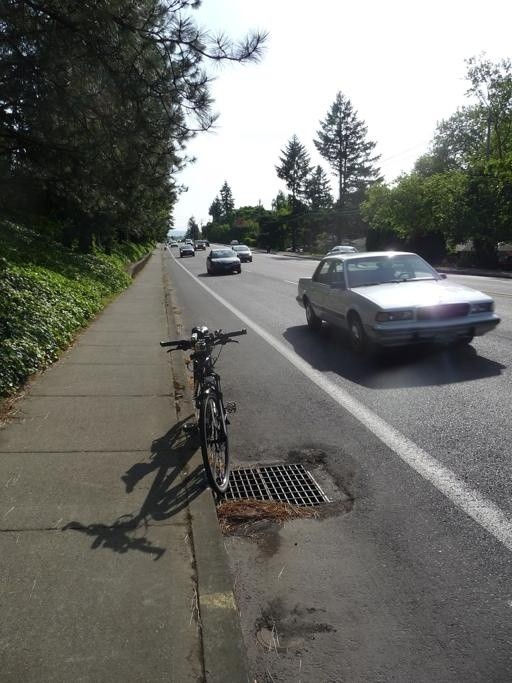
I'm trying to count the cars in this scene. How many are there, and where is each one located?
[326,244,360,267]
[295,250,499,356]
[232,240,239,245]
[232,244,252,263]
[205,248,241,275]
[168,237,210,258]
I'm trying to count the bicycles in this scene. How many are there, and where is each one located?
[159,324,248,493]
